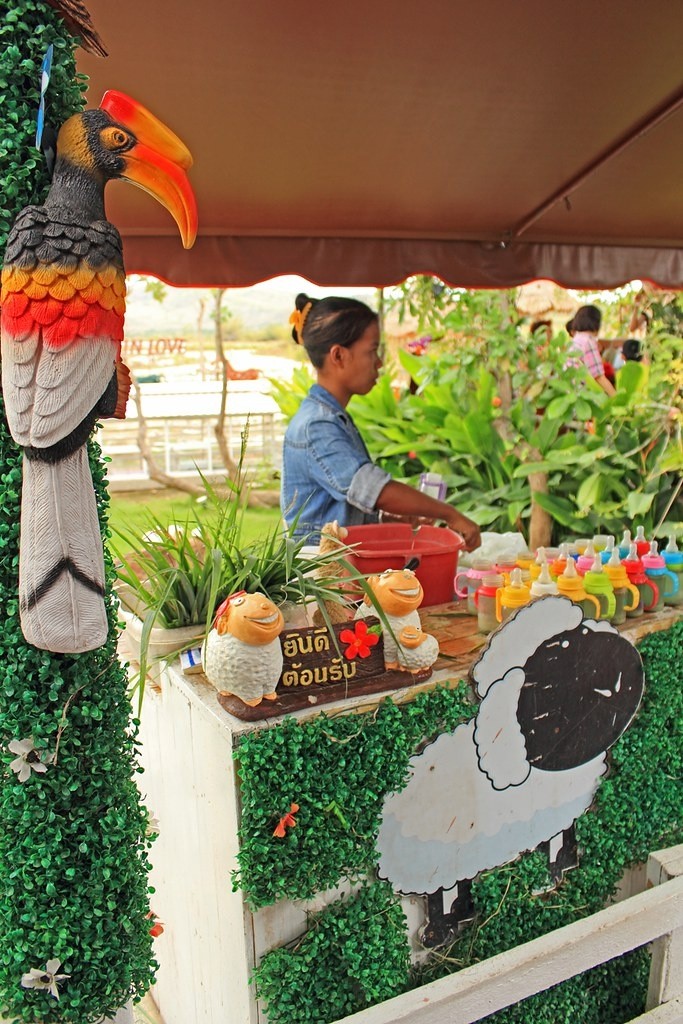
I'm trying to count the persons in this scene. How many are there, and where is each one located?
[281,293,482,583]
[530,305,641,432]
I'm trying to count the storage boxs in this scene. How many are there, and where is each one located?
[110,596,291,689]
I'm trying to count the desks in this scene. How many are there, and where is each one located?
[148,602,683,1023]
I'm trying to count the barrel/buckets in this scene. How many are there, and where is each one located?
[335,520,467,616]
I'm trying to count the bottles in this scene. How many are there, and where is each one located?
[453,526,683,637]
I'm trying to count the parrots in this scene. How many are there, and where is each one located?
[0,88,198,655]
